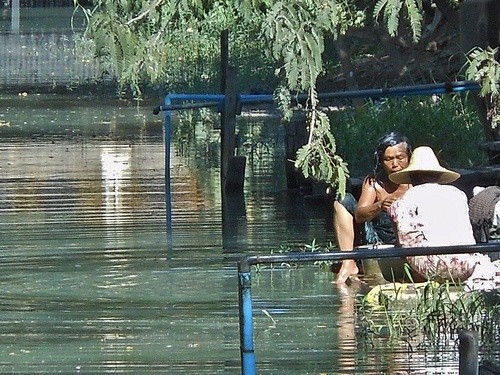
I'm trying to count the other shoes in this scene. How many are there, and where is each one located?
[331,261,364,275]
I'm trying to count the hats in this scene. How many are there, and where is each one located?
[389,146,461,184]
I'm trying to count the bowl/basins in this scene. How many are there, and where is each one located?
[375,257,400,282]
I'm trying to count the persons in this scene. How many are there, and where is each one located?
[387,146,478,283]
[332,131,413,283]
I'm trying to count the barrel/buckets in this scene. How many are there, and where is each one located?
[354,242,396,278]
